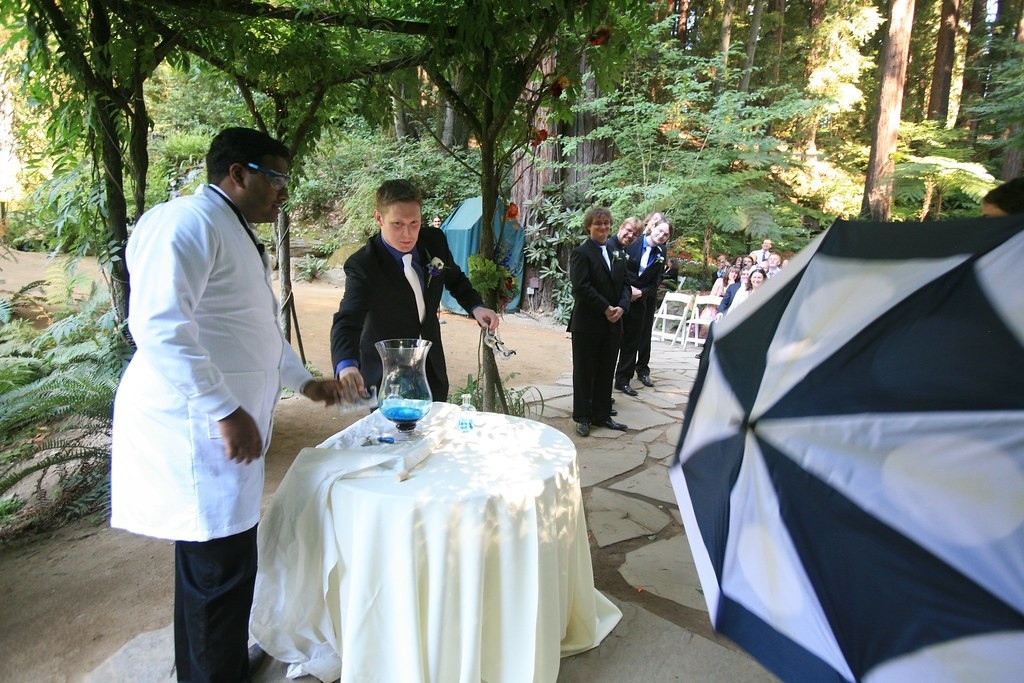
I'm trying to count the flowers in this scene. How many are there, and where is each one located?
[493,244,525,321]
[549,70,589,104]
[528,127,551,146]
[612,249,630,266]
[588,23,613,48]
[423,247,451,288]
[654,254,664,264]
[502,198,520,223]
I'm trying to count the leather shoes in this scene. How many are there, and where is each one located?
[638,375,655,387]
[615,385,638,396]
[576,421,590,436]
[591,418,627,430]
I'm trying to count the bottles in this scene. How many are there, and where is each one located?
[385,385,405,409]
[456,394,476,433]
[374,339,432,440]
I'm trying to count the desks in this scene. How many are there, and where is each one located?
[244,398,624,683]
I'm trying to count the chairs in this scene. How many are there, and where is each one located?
[649,291,723,350]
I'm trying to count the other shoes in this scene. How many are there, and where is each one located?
[248,644,265,676]
[609,410,617,415]
[612,398,615,403]
[695,354,700,359]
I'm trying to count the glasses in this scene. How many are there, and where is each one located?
[592,220,609,226]
[249,164,292,190]
[717,260,724,263]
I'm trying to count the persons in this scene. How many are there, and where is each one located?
[979,177,1023,214]
[331,179,499,416]
[695,239,789,358]
[111,127,345,682]
[606,217,645,415]
[433,215,443,228]
[638,212,665,237]
[658,257,680,310]
[625,219,673,386]
[567,207,628,436]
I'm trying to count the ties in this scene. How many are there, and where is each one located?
[762,252,765,261]
[638,246,651,277]
[601,245,611,269]
[622,246,625,253]
[402,254,426,323]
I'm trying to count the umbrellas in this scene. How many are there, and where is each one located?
[669,214,1024,683]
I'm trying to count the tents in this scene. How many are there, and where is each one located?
[440,195,525,317]
